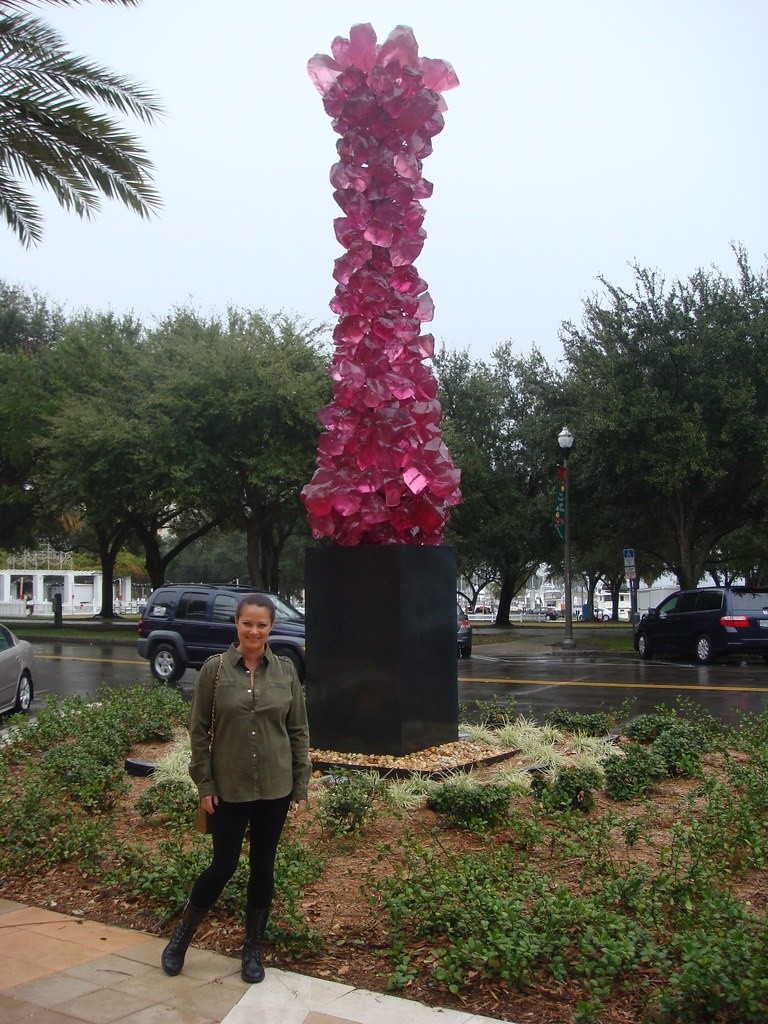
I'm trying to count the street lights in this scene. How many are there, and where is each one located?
[559,427,576,649]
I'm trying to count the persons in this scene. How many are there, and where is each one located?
[161,594,309,983]
[26,594,34,616]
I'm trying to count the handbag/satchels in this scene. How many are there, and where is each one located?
[196,802,211,833]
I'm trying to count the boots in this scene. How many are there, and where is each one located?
[241,919,265,983]
[161,919,197,976]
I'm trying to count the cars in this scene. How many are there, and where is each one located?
[466,606,492,615]
[578,608,614,620]
[1,622,32,715]
[457,602,472,657]
[545,610,565,620]
[635,586,768,662]
[137,583,305,683]
[533,606,550,613]
